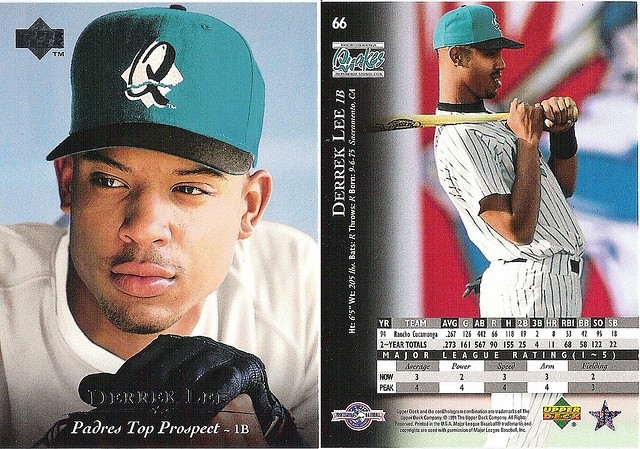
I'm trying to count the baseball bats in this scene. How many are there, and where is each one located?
[344,106,578,135]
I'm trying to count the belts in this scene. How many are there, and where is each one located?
[506,257,580,274]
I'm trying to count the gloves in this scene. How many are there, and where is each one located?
[79,334,310,447]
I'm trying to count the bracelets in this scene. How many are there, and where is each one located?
[549,124,578,159]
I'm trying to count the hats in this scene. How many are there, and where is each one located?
[433,4,525,50]
[46,4,266,176]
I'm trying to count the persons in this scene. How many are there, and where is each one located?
[0,5,320,449]
[431,4,586,319]
